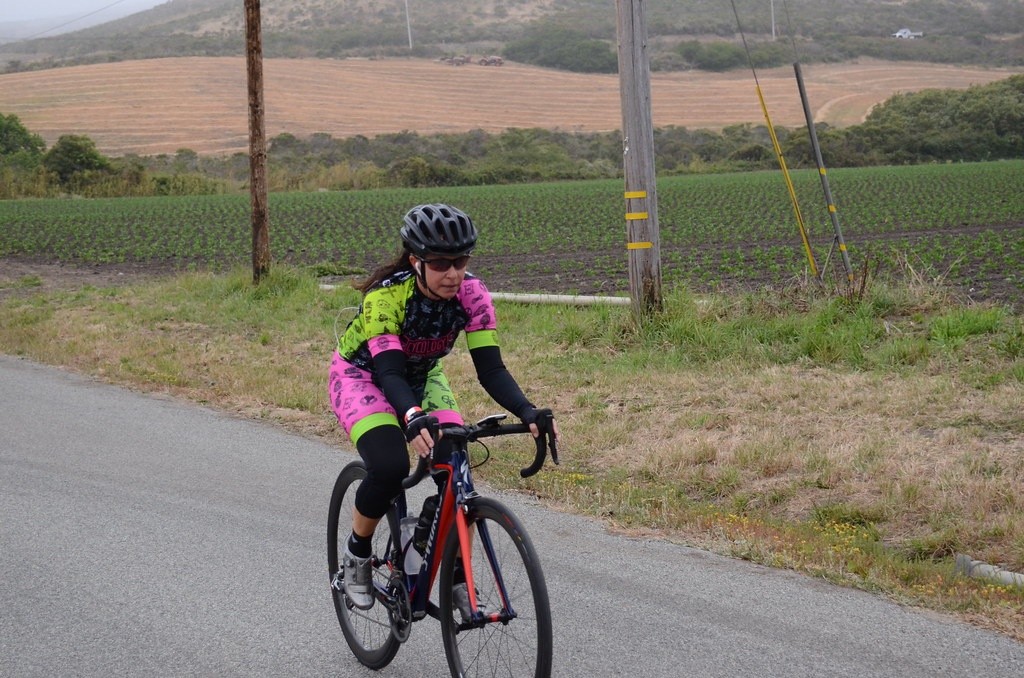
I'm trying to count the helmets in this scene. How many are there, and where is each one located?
[398,203,477,256]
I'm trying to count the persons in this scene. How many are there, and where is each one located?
[328,201,561,630]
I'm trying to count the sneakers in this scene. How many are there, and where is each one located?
[344,533,374,609]
[452,582,486,623]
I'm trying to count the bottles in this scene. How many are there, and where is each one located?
[412,492,439,552]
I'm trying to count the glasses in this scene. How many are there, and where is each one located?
[409,251,471,272]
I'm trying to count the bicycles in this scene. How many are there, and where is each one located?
[322,406,557,677]
